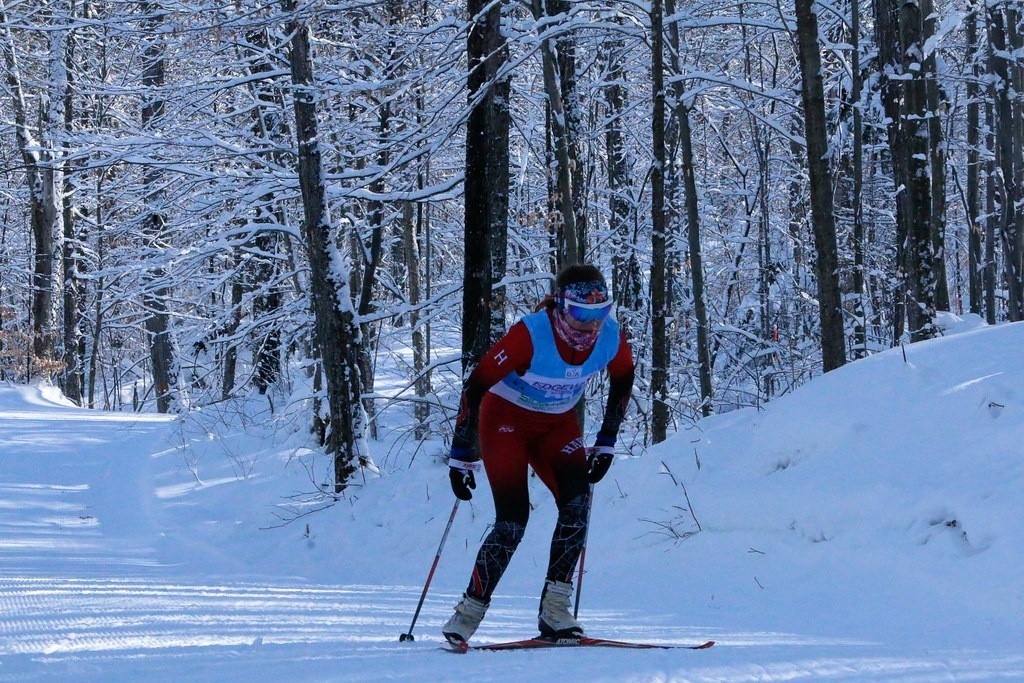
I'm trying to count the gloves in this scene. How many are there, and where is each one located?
[449,467,477,500]
[588,440,614,483]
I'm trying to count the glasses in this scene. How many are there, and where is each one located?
[564,295,614,323]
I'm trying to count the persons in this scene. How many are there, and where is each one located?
[441,263,635,645]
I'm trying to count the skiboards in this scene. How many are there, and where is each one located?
[437,634,719,654]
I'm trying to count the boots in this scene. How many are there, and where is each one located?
[538,577,584,635]
[442,587,491,646]
